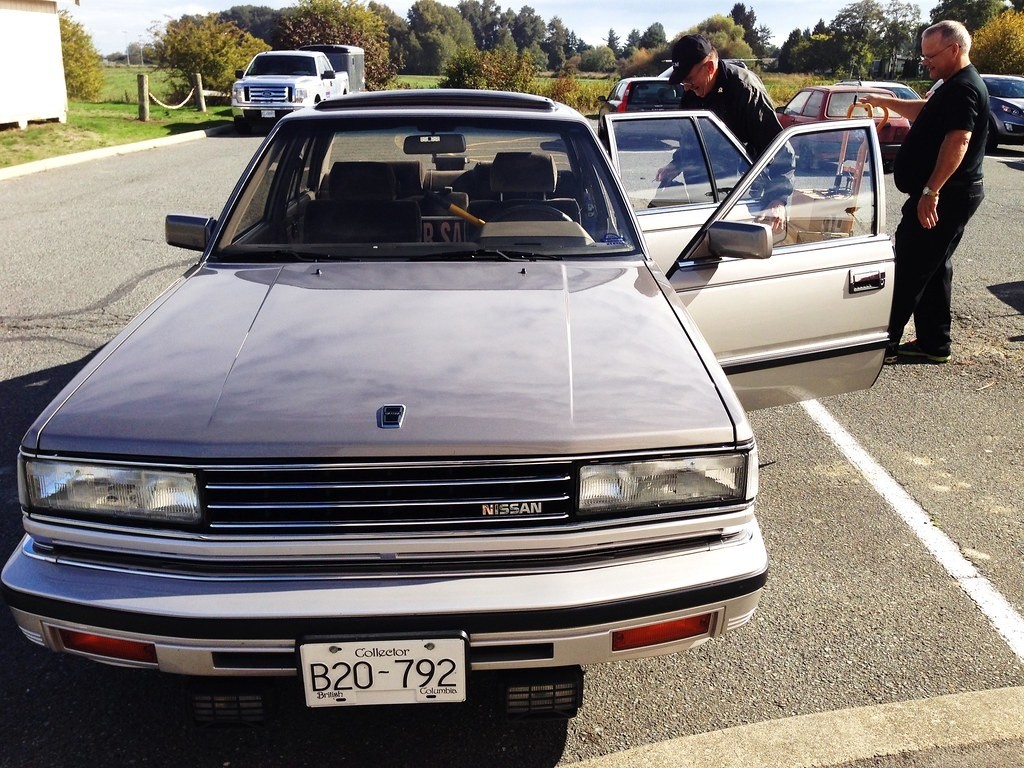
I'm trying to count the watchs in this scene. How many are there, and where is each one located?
[923,187,939,197]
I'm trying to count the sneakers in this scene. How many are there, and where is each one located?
[883,355,897,363]
[897,338,952,361]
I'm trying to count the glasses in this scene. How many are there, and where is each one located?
[920,44,962,62]
[679,63,706,86]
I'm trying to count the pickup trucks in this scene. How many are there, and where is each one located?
[233,50,349,135]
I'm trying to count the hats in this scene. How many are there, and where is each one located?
[668,34,711,85]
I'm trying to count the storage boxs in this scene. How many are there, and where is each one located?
[787,188,853,245]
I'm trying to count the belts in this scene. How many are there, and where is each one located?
[944,180,982,188]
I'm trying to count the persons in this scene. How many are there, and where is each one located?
[655,34,796,236]
[858,20,989,364]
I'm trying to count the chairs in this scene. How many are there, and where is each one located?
[303,151,597,243]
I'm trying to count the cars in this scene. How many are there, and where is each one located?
[0,88,901,725]
[596,77,691,143]
[832,81,923,126]
[775,86,910,174]
[927,75,1024,151]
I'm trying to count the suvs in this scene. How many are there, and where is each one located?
[657,61,750,83]
